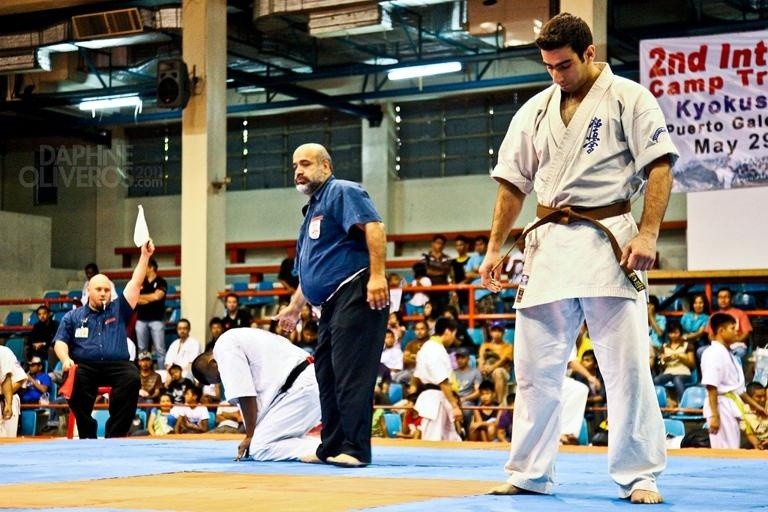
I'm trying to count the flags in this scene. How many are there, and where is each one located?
[132,203,151,248]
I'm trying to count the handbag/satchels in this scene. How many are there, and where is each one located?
[752,342,768,387]
[38,392,50,416]
[146,409,174,435]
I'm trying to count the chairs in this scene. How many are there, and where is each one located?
[227,283,249,306]
[65,290,84,308]
[34,286,61,312]
[19,306,43,336]
[464,324,485,346]
[252,281,279,309]
[18,410,39,437]
[90,408,109,436]
[383,411,404,436]
[160,284,179,305]
[0,308,27,334]
[162,308,184,331]
[399,327,415,348]
[4,337,26,363]
[387,381,406,406]
[505,326,516,350]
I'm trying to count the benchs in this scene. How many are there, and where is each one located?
[116,242,180,264]
[388,223,522,251]
[224,237,297,259]
[93,262,181,282]
[225,261,287,279]
[382,255,427,274]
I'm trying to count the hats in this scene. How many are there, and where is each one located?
[490,320,505,333]
[138,351,153,361]
[28,356,42,364]
[454,347,470,357]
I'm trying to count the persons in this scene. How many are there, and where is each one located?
[275,143,390,469]
[189,326,322,462]
[480,11,684,506]
[1,232,768,450]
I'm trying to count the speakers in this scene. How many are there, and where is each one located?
[155,58,192,108]
